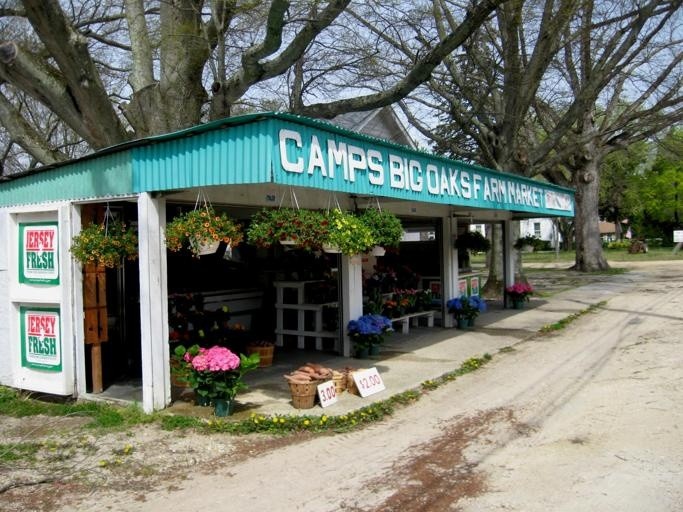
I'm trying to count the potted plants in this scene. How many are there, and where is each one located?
[453,231,545,256]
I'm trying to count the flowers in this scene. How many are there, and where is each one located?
[67,222,138,270]
[506,283,533,303]
[165,209,402,260]
[385,287,436,312]
[169,344,259,398]
[346,313,393,347]
[445,296,486,318]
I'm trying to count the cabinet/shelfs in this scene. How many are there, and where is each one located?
[274,279,436,351]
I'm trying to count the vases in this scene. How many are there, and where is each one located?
[353,345,381,360]
[513,301,522,309]
[458,317,476,328]
[194,388,235,416]
